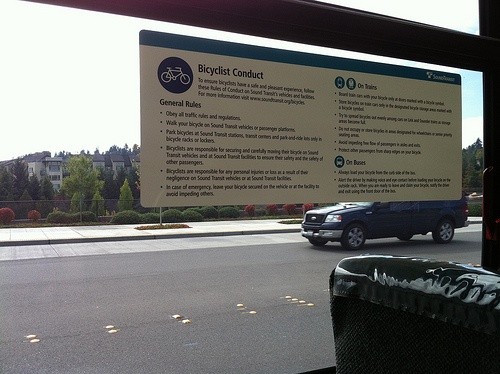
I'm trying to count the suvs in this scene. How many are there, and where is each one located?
[301,194,470,252]
